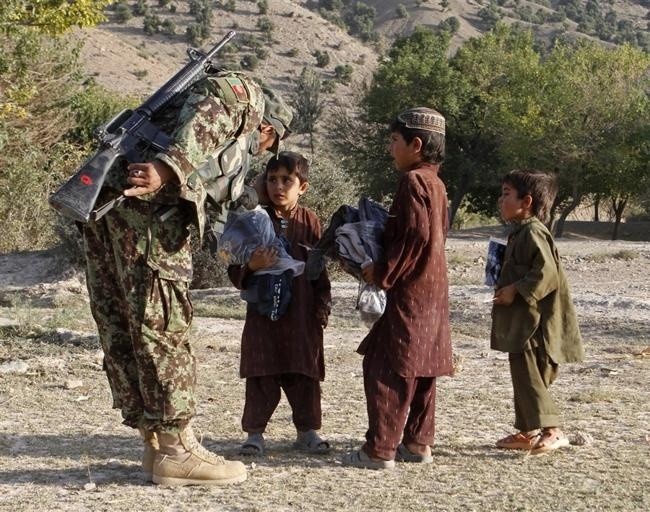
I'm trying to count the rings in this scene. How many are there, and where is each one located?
[133,170,141,177]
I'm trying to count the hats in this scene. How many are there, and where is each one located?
[398,107,445,137]
[260,87,294,160]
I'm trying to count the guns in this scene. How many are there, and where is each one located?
[47,28,237,224]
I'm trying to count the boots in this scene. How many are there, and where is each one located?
[152,426,247,486]
[141,431,160,481]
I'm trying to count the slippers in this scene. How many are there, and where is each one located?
[531,431,569,453]
[241,437,264,455]
[395,444,434,463]
[496,433,541,448]
[293,435,331,454]
[342,452,395,469]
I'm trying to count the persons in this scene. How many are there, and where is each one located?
[323,106,454,471]
[79,71,294,488]
[489,168,586,453]
[226,150,334,457]
